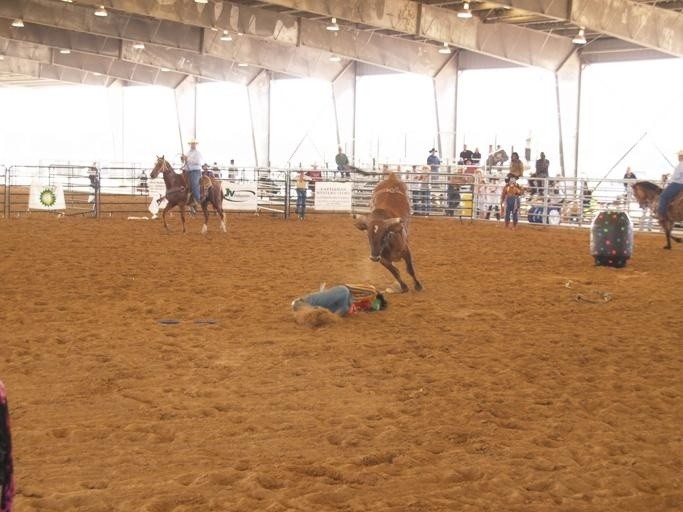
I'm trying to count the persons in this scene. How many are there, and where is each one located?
[380,142,592,228]
[658,151,683,217]
[335,146,351,179]
[201,163,214,179]
[306,165,323,189]
[227,160,239,184]
[623,167,637,199]
[291,284,388,324]
[139,169,148,196]
[659,175,670,187]
[181,139,202,212]
[296,165,311,220]
[211,162,222,178]
[89,162,99,193]
[0,380,16,512]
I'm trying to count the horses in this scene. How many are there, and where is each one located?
[629,181,683,249]
[150,154,227,234]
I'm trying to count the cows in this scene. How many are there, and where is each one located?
[342,164,423,293]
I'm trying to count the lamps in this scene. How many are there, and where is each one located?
[94,5,108,17]
[324,16,340,32]
[11,16,25,29]
[455,0,473,19]
[571,25,587,46]
[132,41,144,49]
[219,30,232,43]
[328,54,340,63]
[437,42,453,55]
